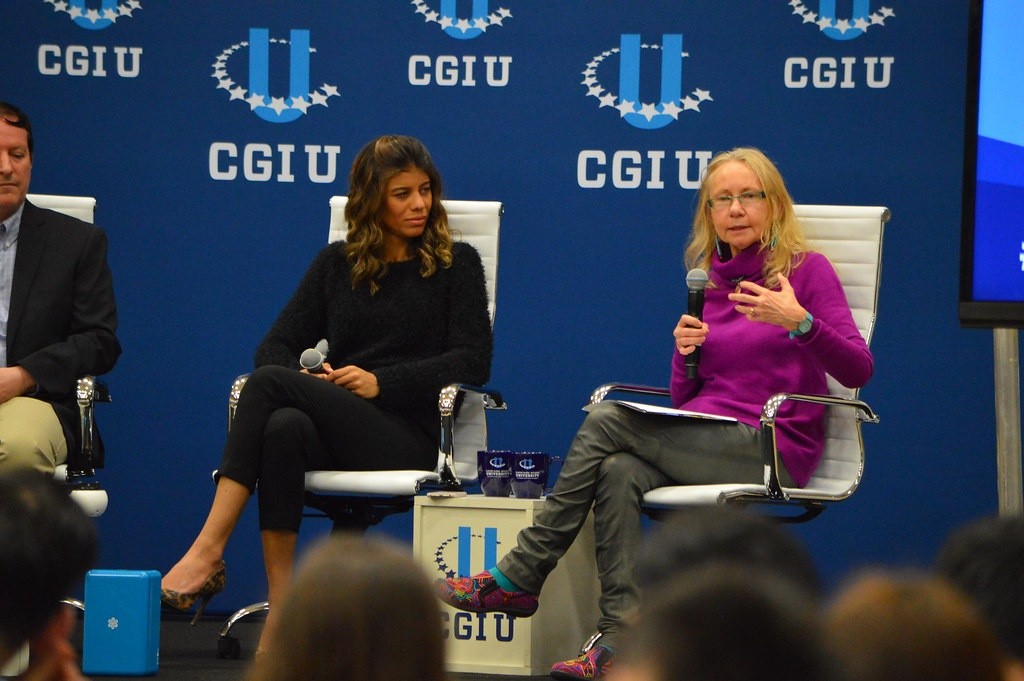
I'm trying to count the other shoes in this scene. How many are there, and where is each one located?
[550,644,618,681]
[434,571,538,618]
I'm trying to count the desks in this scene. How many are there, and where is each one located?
[411,492,604,676]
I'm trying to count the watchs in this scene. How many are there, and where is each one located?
[788,314,814,341]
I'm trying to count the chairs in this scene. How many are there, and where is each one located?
[24,195,109,518]
[577,201,889,523]
[209,194,505,666]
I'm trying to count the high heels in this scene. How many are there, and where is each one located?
[161,560,225,626]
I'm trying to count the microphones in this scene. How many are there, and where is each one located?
[684,268,708,379]
[300,348,324,375]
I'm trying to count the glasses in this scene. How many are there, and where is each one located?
[707,191,767,207]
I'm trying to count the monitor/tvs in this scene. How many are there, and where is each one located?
[957,0,1024,329]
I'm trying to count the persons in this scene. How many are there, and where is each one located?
[618,503,1024,681]
[255,534,445,680]
[159,137,491,672]
[0,98,120,473]
[436,147,871,681]
[1,467,99,681]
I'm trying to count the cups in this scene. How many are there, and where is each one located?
[511,451,565,498]
[477,450,514,498]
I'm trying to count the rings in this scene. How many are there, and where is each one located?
[750,308,755,316]
